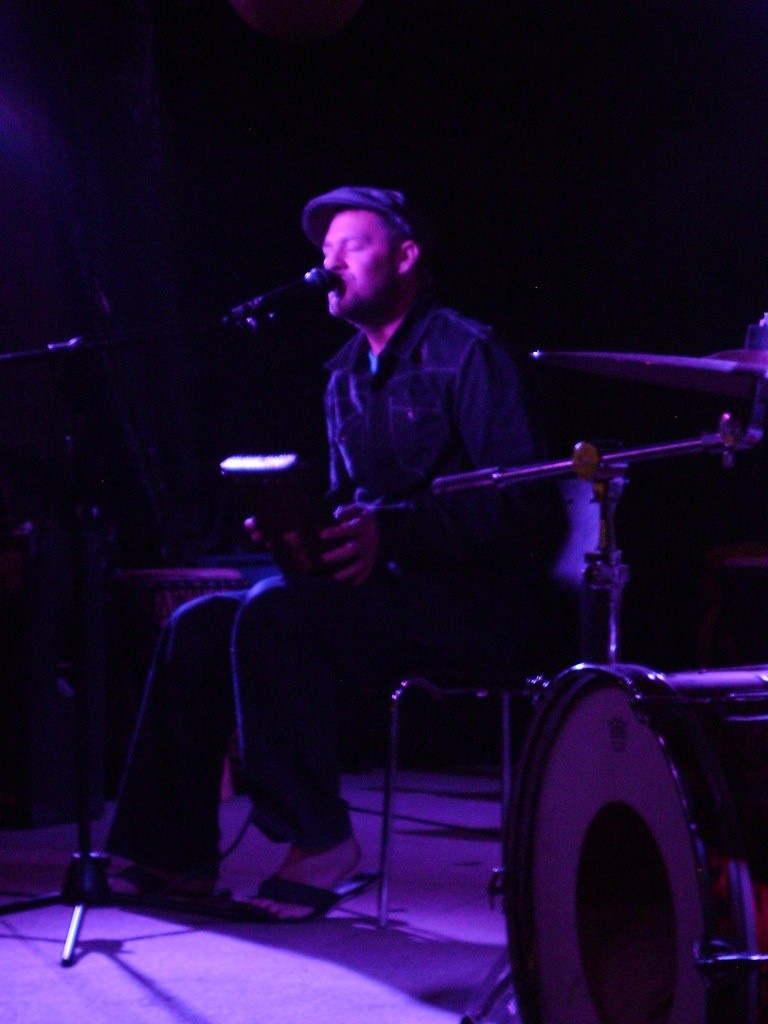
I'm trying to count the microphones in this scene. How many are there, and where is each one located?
[695,941,738,980]
[231,267,337,319]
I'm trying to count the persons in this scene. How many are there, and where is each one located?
[62,187,547,926]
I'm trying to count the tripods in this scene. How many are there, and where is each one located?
[0,313,279,966]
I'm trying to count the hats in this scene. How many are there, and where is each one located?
[301,186,422,241]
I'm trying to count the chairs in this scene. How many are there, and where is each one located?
[348,476,619,931]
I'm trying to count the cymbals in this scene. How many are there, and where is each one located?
[530,348,768,400]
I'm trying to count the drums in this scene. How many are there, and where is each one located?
[503,663,768,1024]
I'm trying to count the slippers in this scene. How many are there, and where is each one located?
[239,866,384,923]
[106,866,244,919]
